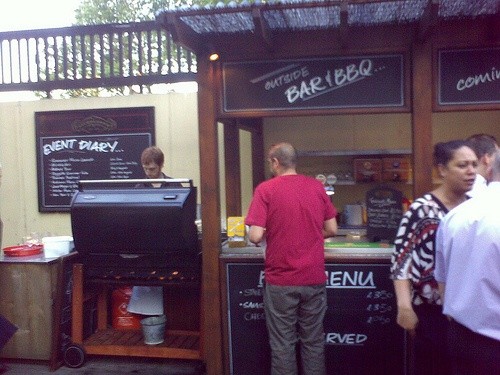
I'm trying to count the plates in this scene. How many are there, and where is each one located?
[2,244,44,257]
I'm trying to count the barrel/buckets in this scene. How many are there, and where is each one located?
[111,289,142,330]
[126,285,164,316]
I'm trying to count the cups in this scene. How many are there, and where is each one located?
[140,316,167,345]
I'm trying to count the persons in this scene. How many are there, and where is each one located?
[244,142,339,374]
[388,138,480,375]
[133,145,184,191]
[459,133,500,199]
[434,147,500,375]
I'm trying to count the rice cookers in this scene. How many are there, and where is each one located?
[344,205,363,226]
